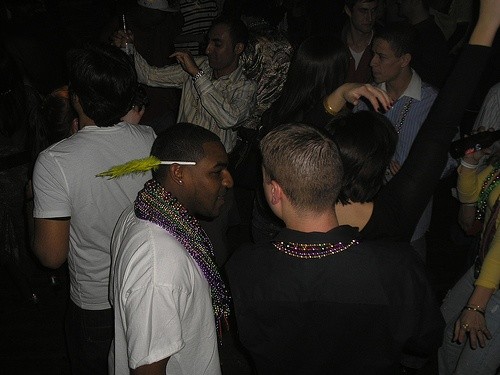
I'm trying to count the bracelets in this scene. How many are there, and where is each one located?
[462,303,485,315]
[192,70,204,82]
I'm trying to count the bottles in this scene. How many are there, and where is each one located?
[449,130,500,158]
[118,9,134,55]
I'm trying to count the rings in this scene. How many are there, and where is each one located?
[460,321,469,331]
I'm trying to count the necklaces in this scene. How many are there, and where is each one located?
[272,236,361,257]
[472,167,500,282]
[134,179,232,345]
[394,97,413,135]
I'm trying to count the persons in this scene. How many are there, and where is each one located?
[108,122,235,375]
[0,0,500,375]
